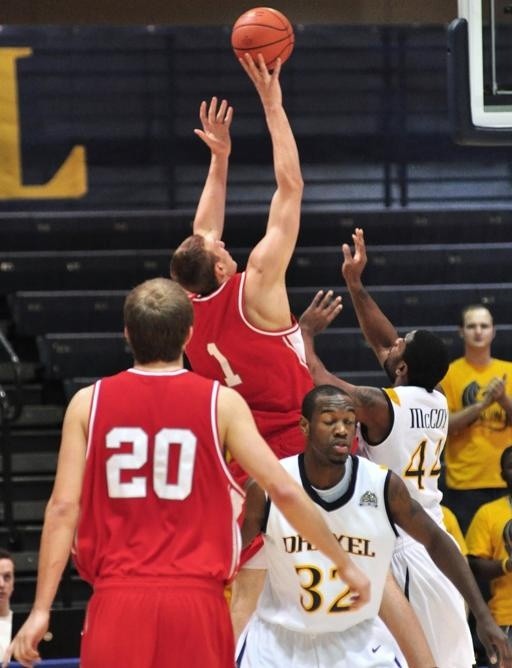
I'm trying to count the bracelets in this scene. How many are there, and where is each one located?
[500,555,509,577]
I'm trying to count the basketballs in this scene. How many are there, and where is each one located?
[232,6,295,70]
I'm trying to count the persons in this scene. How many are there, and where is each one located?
[0,549,28,667]
[0,277,371,667]
[223,383,511,668]
[468,447,511,666]
[434,301,511,519]
[299,224,481,668]
[168,52,360,651]
[437,495,472,612]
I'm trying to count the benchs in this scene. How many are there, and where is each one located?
[0,19,512,420]
